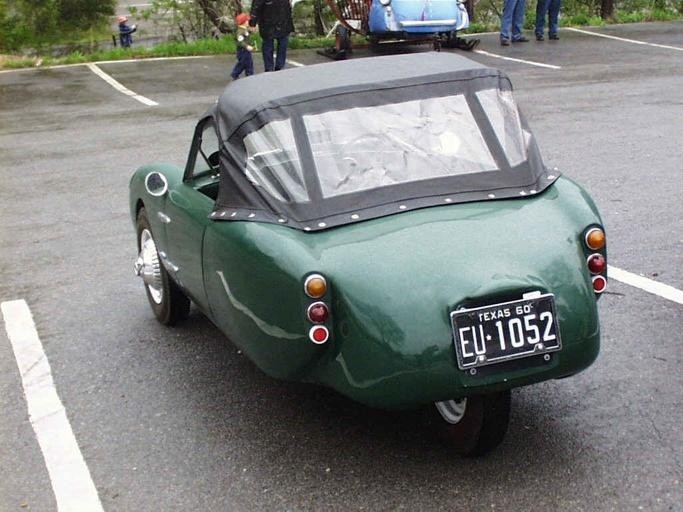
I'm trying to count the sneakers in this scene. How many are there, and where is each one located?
[501,38,511,45]
[230,75,240,81]
[512,36,530,42]
[536,35,545,42]
[549,33,560,40]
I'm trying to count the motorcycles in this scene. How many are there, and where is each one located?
[126,49,608,456]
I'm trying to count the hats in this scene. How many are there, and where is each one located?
[118,17,127,24]
[236,13,253,26]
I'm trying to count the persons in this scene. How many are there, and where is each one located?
[247,1,296,73]
[116,15,139,50]
[534,1,561,41]
[228,13,257,80]
[498,0,530,46]
